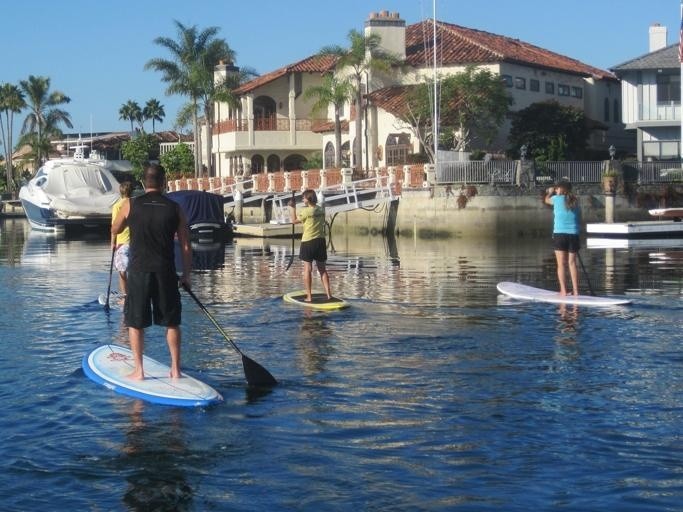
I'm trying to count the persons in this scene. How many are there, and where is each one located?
[544,181,581,296]
[287,190,332,302]
[110,164,191,381]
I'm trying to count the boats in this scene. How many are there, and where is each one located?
[495,281,633,306]
[18,158,122,232]
[167,190,227,236]
[98,291,122,310]
[81,344,224,409]
[283,289,352,311]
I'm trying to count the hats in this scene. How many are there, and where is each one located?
[556,181,572,191]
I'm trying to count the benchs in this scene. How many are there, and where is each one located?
[648,206,683,222]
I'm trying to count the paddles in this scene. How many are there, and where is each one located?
[286,190,295,268]
[177,274,278,386]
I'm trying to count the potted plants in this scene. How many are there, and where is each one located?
[600,166,624,196]
